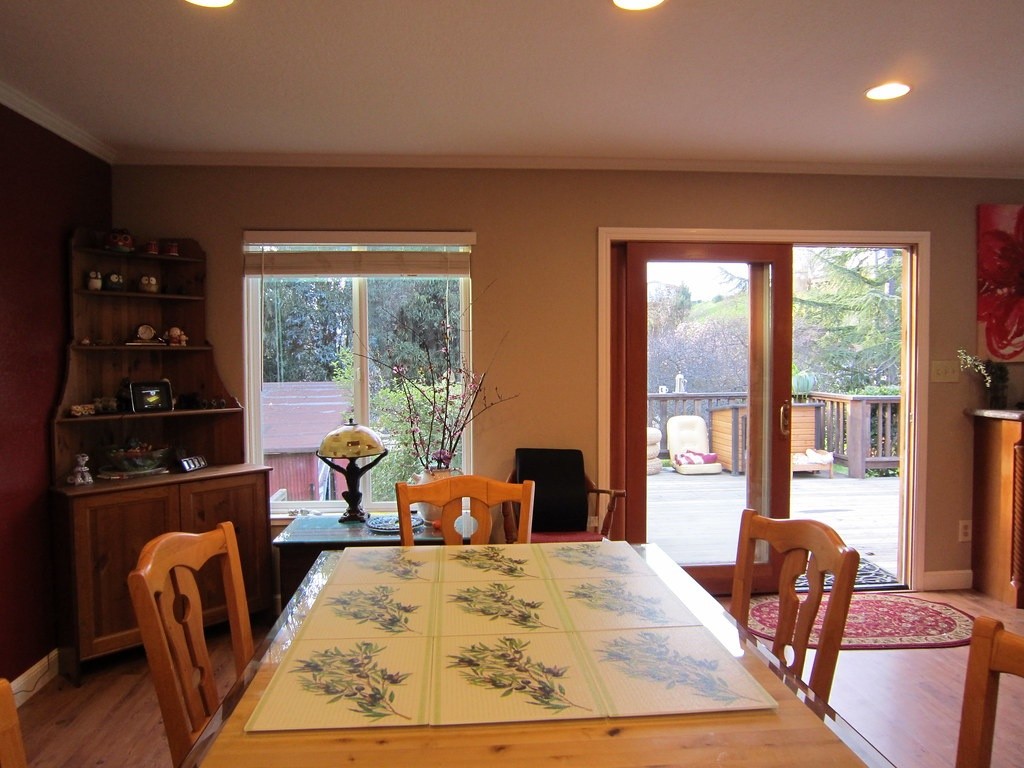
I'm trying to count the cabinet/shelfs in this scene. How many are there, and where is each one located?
[964,407,1024,608]
[48,225,273,687]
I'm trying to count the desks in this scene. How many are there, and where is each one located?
[181,542,896,768]
[273,512,470,619]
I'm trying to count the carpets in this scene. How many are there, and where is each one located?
[794,558,898,586]
[746,593,976,650]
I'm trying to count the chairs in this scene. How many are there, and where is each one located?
[128,521,255,768]
[956,615,1024,768]
[502,448,626,544]
[667,415,722,474]
[730,509,860,721]
[396,475,535,546]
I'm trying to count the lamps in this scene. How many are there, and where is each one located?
[316,419,389,522]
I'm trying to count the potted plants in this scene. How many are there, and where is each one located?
[957,350,1009,410]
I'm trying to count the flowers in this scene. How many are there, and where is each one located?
[353,283,521,479]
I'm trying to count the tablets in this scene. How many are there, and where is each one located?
[130,381,174,413]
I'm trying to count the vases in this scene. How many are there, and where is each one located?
[413,467,464,523]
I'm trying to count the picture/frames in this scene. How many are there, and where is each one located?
[129,378,174,412]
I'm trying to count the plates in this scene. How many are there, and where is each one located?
[366,514,424,533]
